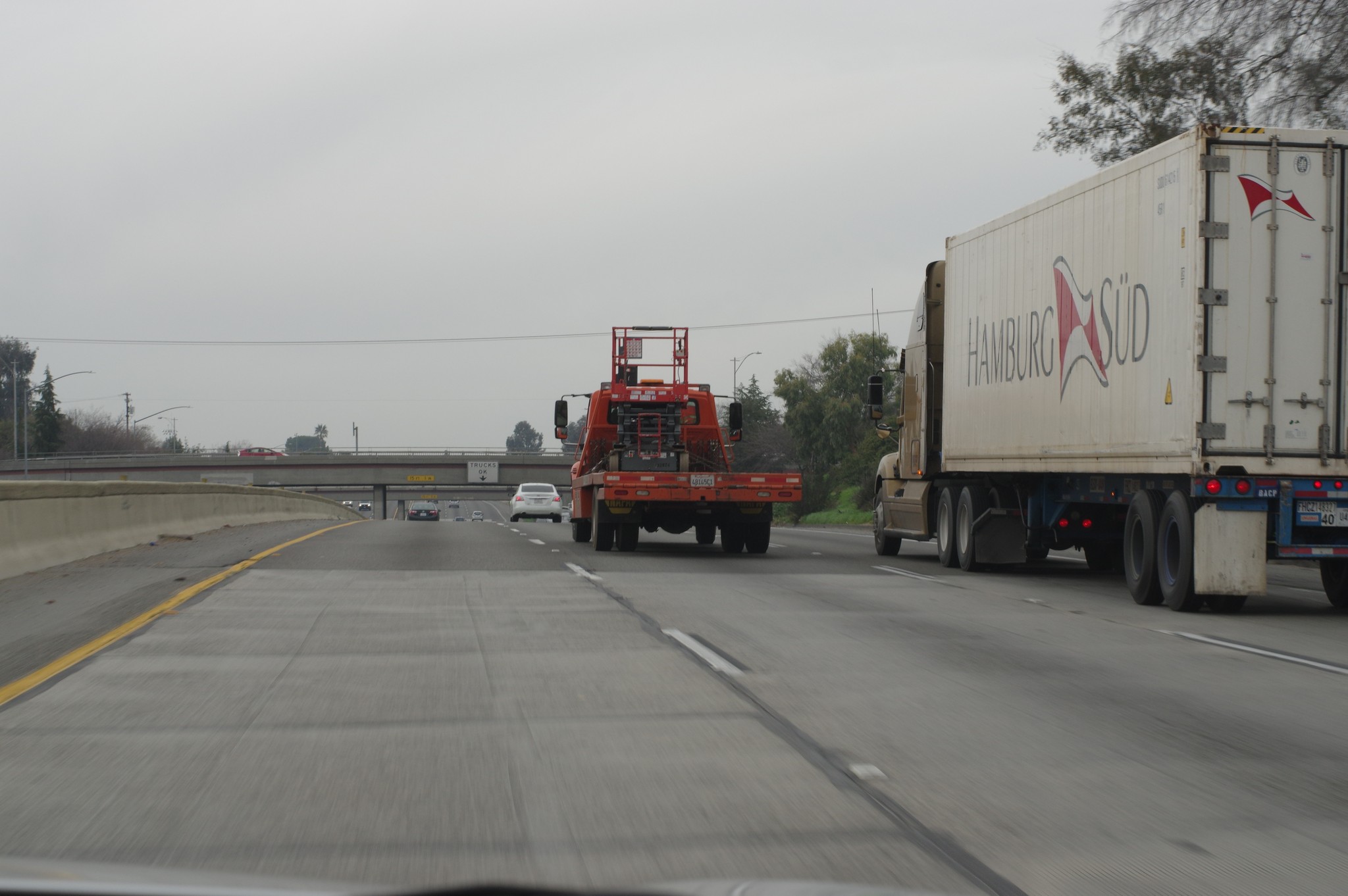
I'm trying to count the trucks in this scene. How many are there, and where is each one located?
[553,327,802,554]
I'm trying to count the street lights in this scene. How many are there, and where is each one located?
[734,352,762,402]
[23,371,96,479]
[158,417,176,454]
[132,406,191,454]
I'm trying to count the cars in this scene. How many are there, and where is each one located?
[343,501,353,507]
[237,447,288,456]
[508,483,573,522]
[405,502,441,521]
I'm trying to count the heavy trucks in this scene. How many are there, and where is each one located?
[868,122,1347,615]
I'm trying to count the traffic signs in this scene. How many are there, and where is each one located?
[466,460,499,484]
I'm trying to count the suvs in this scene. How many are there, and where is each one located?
[471,511,484,521]
[449,500,460,508]
[453,516,466,522]
[358,501,372,511]
